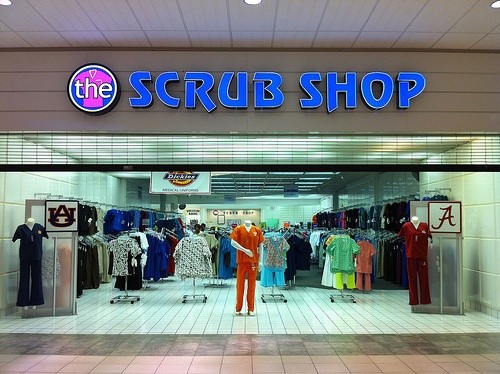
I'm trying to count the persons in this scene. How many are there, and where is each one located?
[12,218,49,310]
[230,220,264,316]
[398,216,434,305]
